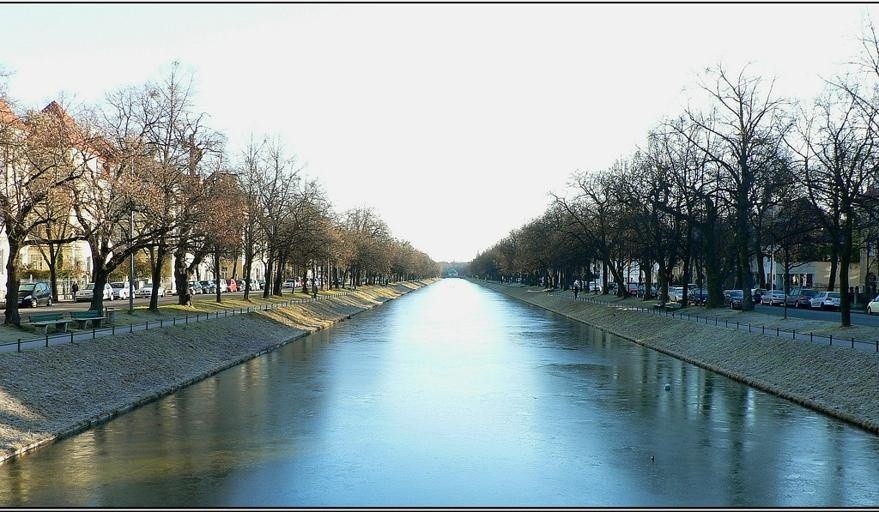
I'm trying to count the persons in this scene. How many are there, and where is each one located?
[71,281,78,299]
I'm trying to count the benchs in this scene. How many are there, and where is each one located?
[28,311,109,334]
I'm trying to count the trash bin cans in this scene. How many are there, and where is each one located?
[106,307,114,324]
[675,296,678,302]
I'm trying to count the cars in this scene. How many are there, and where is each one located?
[574,279,841,311]
[18,280,53,308]
[866,293,879,315]
[74,276,318,303]
